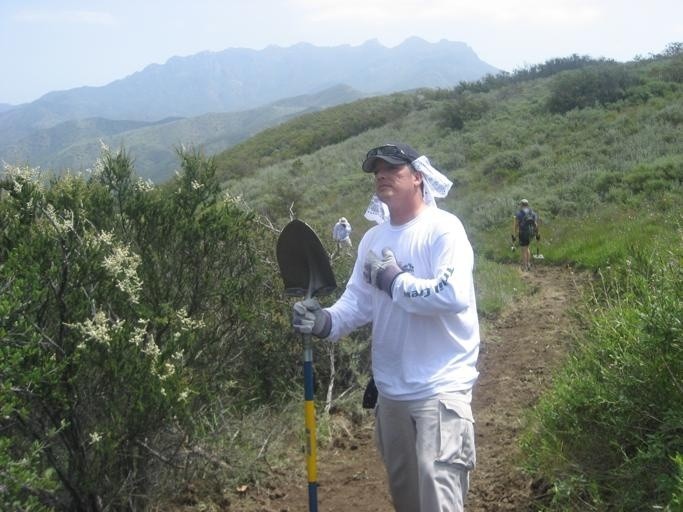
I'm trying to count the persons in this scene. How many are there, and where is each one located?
[333,217,353,257]
[292,145,481,512]
[512,199,540,272]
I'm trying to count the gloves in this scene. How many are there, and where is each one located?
[292,299,332,339]
[362,249,403,298]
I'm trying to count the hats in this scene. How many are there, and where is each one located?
[339,217,346,224]
[362,144,418,172]
[518,198,528,206]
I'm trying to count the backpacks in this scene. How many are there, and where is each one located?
[520,209,535,238]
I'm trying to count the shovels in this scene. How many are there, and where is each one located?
[511,215,516,252]
[276,218,338,512]
[533,240,544,259]
